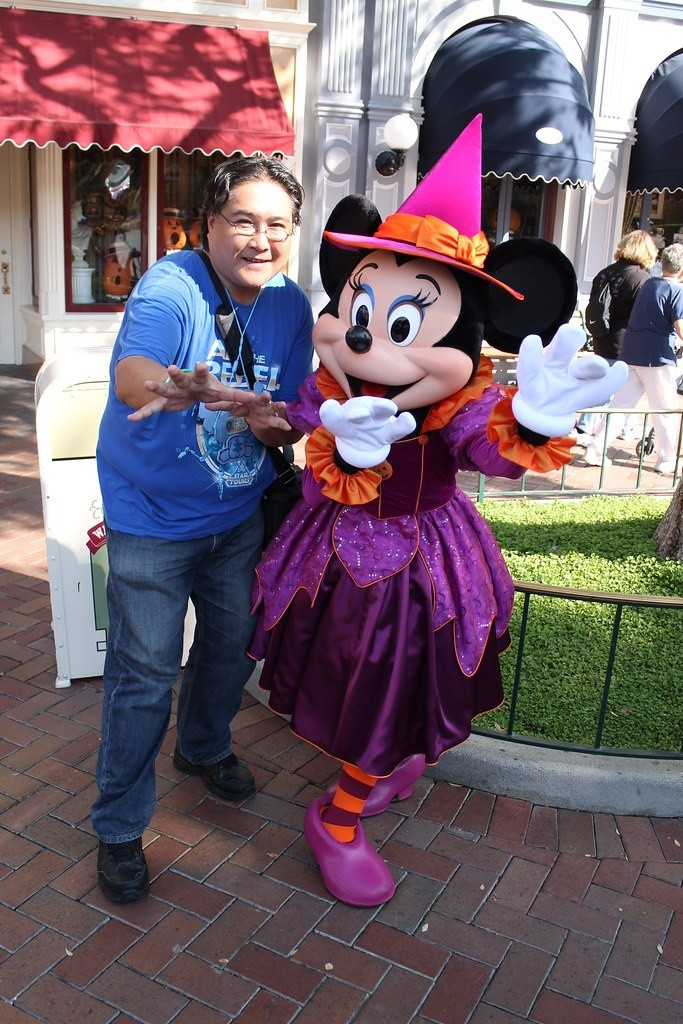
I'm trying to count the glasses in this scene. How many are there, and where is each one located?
[219,210,296,242]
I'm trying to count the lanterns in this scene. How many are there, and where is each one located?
[103,205,207,303]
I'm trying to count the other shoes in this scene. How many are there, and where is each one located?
[585,450,613,467]
[655,460,683,474]
[572,428,593,447]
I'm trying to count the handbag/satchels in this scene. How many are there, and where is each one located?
[262,465,305,549]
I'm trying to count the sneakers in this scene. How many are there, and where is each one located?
[173,747,255,800]
[97,836,149,903]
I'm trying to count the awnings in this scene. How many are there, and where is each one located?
[0,6,297,158]
[417,14,596,191]
[625,52,683,197]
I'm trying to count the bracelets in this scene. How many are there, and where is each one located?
[165,369,193,384]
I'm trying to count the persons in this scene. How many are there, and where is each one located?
[570,230,683,473]
[90,155,316,905]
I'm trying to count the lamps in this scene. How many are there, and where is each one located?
[375,115,419,178]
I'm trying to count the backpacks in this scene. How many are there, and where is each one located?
[585,265,635,337]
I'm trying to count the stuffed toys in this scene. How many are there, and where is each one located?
[243,112,631,907]
